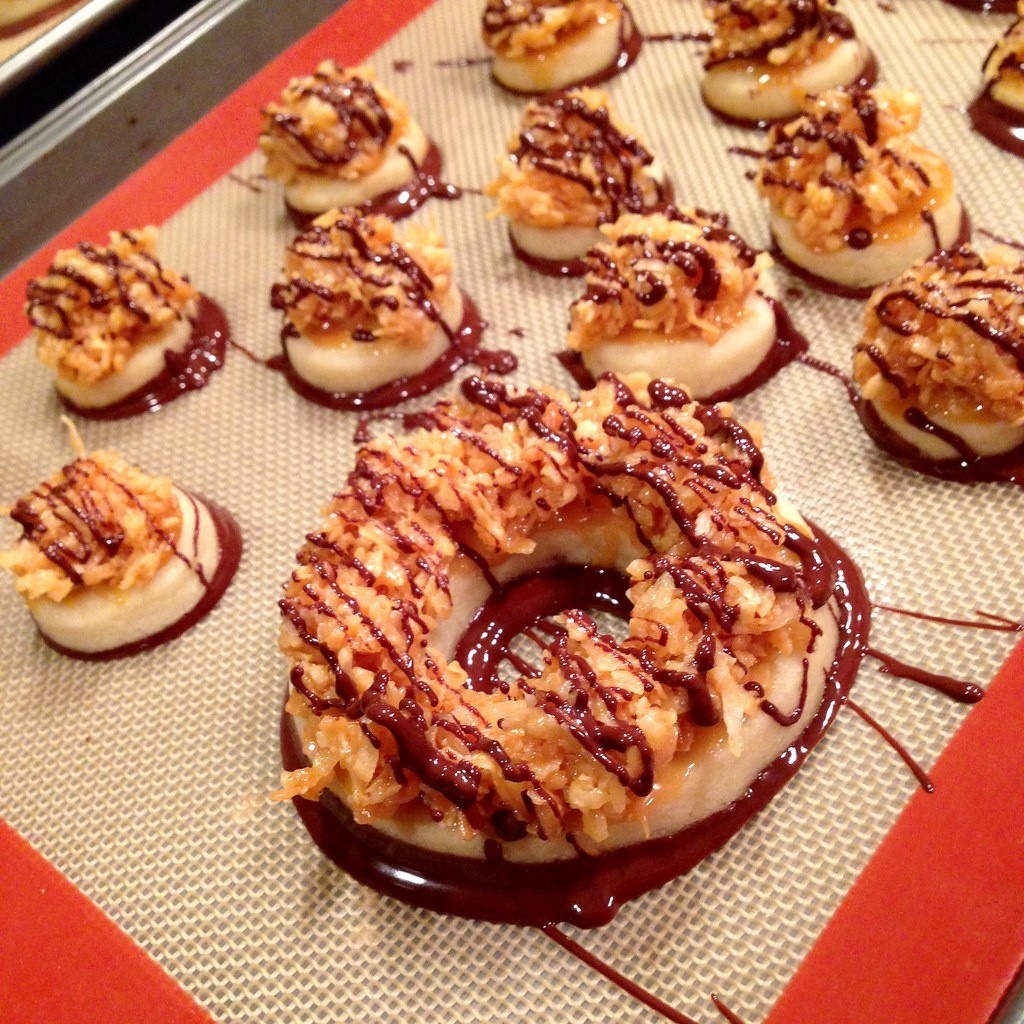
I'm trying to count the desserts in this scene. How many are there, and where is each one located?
[0,0,1024,862]
